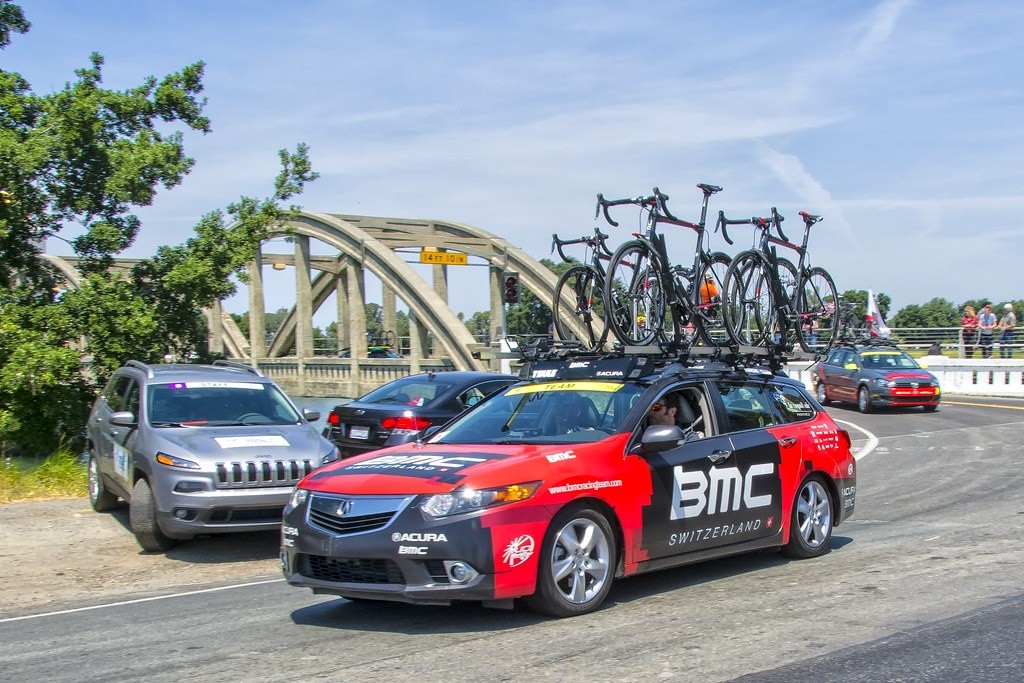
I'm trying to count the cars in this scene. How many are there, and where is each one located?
[280,345,855,617]
[812,337,940,413]
[339,347,403,358]
[321,371,518,459]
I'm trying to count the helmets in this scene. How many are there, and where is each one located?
[1003,303,1013,312]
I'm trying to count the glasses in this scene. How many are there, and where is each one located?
[652,404,666,412]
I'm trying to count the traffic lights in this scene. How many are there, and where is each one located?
[500,271,519,303]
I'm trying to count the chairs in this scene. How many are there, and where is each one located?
[675,395,705,438]
[582,396,612,435]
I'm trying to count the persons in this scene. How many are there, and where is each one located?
[521,392,595,438]
[862,355,892,368]
[549,323,555,339]
[700,274,720,317]
[774,316,819,353]
[586,393,699,450]
[961,302,1015,359]
[685,322,694,348]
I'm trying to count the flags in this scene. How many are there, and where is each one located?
[867,291,892,340]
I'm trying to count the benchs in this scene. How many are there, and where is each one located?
[726,406,782,430]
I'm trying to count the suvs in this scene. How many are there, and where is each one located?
[88,360,341,551]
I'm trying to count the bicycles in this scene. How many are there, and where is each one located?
[595,183,745,347]
[714,206,840,353]
[367,321,396,346]
[550,228,700,354]
